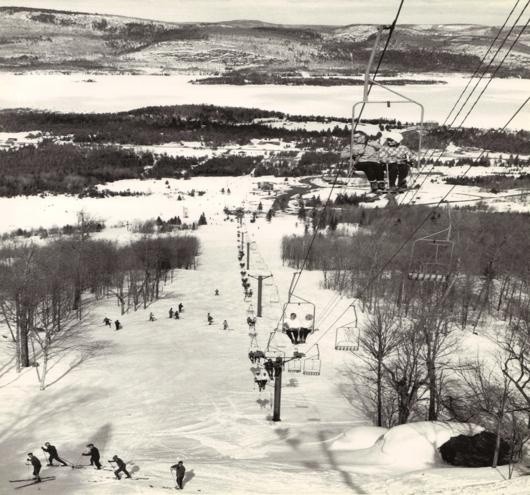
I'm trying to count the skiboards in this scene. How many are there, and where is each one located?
[47,464,72,467]
[149,484,201,491]
[94,464,115,471]
[9,476,55,490]
[77,464,94,467]
[107,475,149,480]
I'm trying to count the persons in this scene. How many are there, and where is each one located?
[215,289,219,295]
[283,312,300,345]
[339,128,386,191]
[179,303,183,312]
[82,444,102,470]
[41,443,68,466]
[149,312,154,320]
[208,312,213,325]
[170,461,185,490]
[249,351,263,363]
[114,320,120,330]
[174,311,179,319]
[168,307,173,318]
[103,317,112,325]
[223,320,228,329]
[27,453,41,482]
[273,357,281,377]
[263,359,273,381]
[297,313,314,343]
[379,131,415,190]
[109,456,131,480]
